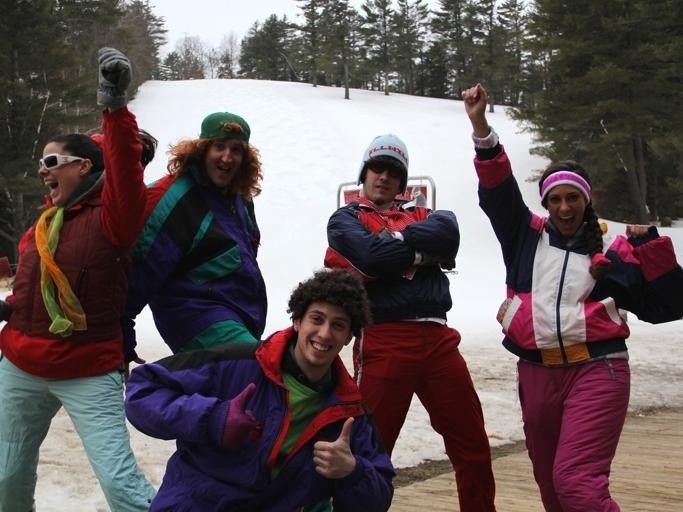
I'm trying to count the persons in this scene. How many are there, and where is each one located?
[119,113,268,387]
[0,46,159,512]
[124,268,397,512]
[324,135,497,511]
[463,82,683,512]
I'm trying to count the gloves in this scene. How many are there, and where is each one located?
[97,46,132,109]
[221,383,261,449]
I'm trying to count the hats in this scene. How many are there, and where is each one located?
[199,111,250,142]
[356,134,409,195]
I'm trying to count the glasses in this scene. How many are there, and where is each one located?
[370,161,401,179]
[38,154,83,170]
